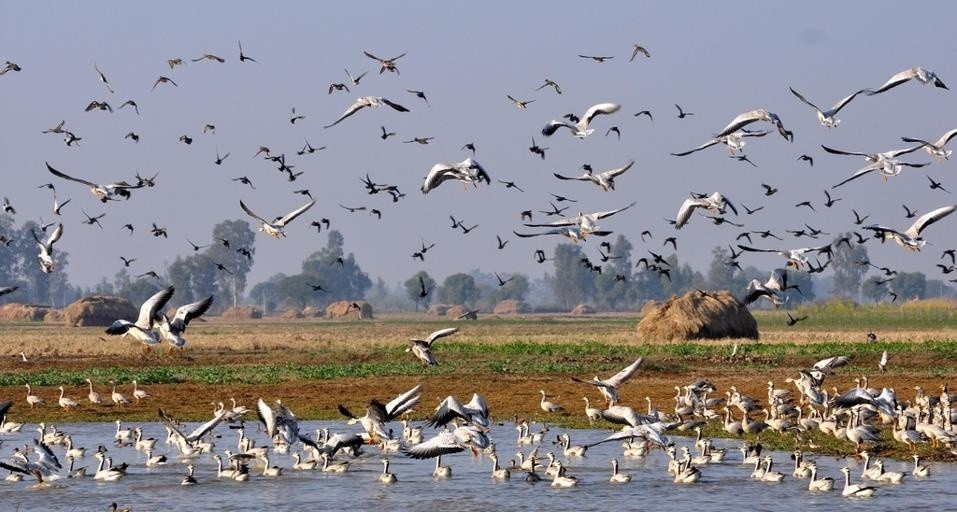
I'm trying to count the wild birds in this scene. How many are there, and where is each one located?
[572,356,644,408]
[812,354,850,375]
[876,349,889,373]
[786,312,808,327]
[153,294,215,355]
[107,503,130,512]
[403,328,458,366]
[0,370,956,498]
[0,41,957,320]
[105,285,174,351]
[868,329,876,340]
